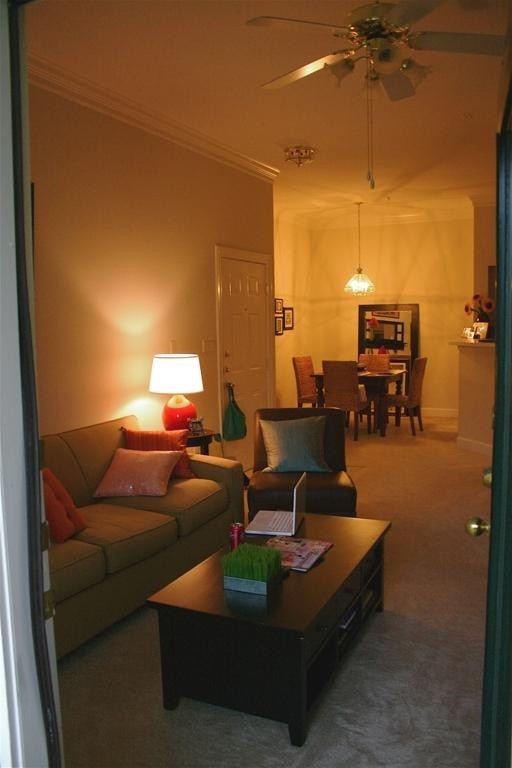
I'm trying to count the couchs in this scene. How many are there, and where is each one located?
[40,414,244,661]
[247,407,357,521]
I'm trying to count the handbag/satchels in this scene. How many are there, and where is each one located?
[223,384,246,441]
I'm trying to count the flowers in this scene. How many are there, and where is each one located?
[463,292,496,315]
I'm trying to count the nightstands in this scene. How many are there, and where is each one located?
[364,319,404,349]
[358,358,410,415]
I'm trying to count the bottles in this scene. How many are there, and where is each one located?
[230,522,244,551]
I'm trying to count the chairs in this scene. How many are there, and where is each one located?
[386,357,427,436]
[359,354,390,424]
[322,360,371,441]
[292,356,318,407]
[387,354,410,358]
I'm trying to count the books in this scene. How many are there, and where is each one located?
[262,537,333,571]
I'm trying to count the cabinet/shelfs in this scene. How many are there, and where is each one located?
[449,342,495,456]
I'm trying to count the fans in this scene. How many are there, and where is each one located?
[246,0,511,102]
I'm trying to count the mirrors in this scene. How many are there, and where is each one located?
[365,310,412,359]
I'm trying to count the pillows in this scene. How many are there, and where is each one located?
[91,448,183,496]
[41,467,89,545]
[121,426,196,479]
[259,417,334,473]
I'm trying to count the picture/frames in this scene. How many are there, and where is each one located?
[467,332,474,339]
[275,298,283,313]
[473,322,488,340]
[275,317,283,335]
[372,311,399,318]
[283,308,294,330]
[462,327,470,337]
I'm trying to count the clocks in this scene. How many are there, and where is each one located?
[187,418,205,436]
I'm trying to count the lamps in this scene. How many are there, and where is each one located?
[343,202,376,297]
[368,311,379,328]
[149,354,203,430]
[285,147,316,165]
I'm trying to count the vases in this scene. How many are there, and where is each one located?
[477,313,492,337]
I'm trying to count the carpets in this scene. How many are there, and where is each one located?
[58,418,494,766]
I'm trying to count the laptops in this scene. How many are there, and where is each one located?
[245,472,307,536]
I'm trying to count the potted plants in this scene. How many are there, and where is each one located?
[221,543,283,595]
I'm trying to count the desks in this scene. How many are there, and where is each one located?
[310,370,405,437]
